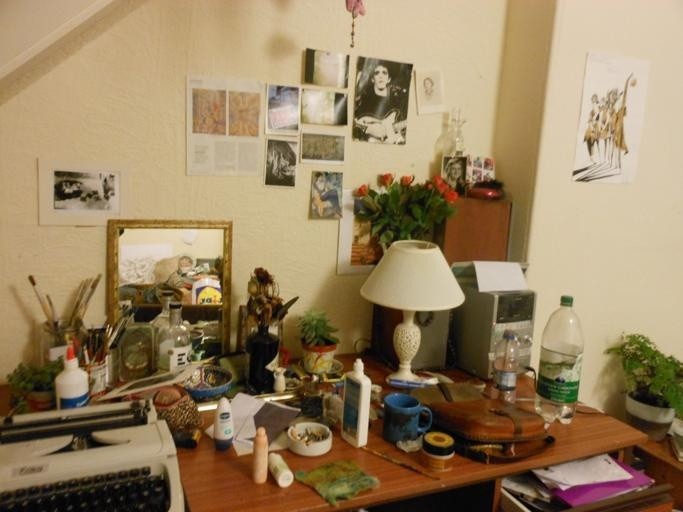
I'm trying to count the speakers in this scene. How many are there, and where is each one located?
[370,303,450,373]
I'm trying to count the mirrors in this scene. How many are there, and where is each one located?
[105,217,233,354]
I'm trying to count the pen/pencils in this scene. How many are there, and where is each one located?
[82,331,104,367]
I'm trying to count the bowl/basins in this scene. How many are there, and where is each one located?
[189,331,203,345]
[182,365,234,402]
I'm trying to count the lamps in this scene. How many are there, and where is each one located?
[359,235,469,388]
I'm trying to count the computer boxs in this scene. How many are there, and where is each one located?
[450,282,539,381]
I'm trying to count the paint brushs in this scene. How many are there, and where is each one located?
[28,275,101,345]
[105,307,139,349]
[362,447,444,481]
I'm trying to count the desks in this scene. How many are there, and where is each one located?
[0,350,674,510]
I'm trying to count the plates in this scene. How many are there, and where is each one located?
[298,355,342,377]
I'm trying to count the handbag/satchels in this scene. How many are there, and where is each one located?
[428,399,554,464]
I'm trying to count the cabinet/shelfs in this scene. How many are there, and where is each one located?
[632,432,681,512]
[435,194,513,270]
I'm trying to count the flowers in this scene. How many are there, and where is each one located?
[352,166,461,254]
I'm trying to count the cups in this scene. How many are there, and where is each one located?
[272,366,287,392]
[40,320,87,364]
[382,392,432,442]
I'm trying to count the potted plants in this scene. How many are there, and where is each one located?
[292,307,343,374]
[601,330,682,444]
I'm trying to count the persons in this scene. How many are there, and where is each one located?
[351,60,407,145]
[313,171,343,217]
[441,157,464,192]
[417,76,440,105]
[162,255,203,290]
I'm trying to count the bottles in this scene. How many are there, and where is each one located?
[156,302,189,371]
[150,292,189,370]
[266,452,294,488]
[489,330,519,404]
[252,427,268,485]
[532,294,585,425]
